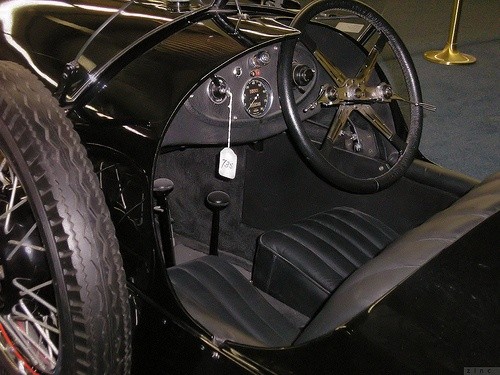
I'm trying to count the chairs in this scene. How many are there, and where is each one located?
[159,190,500,375]
[250,171,500,321]
